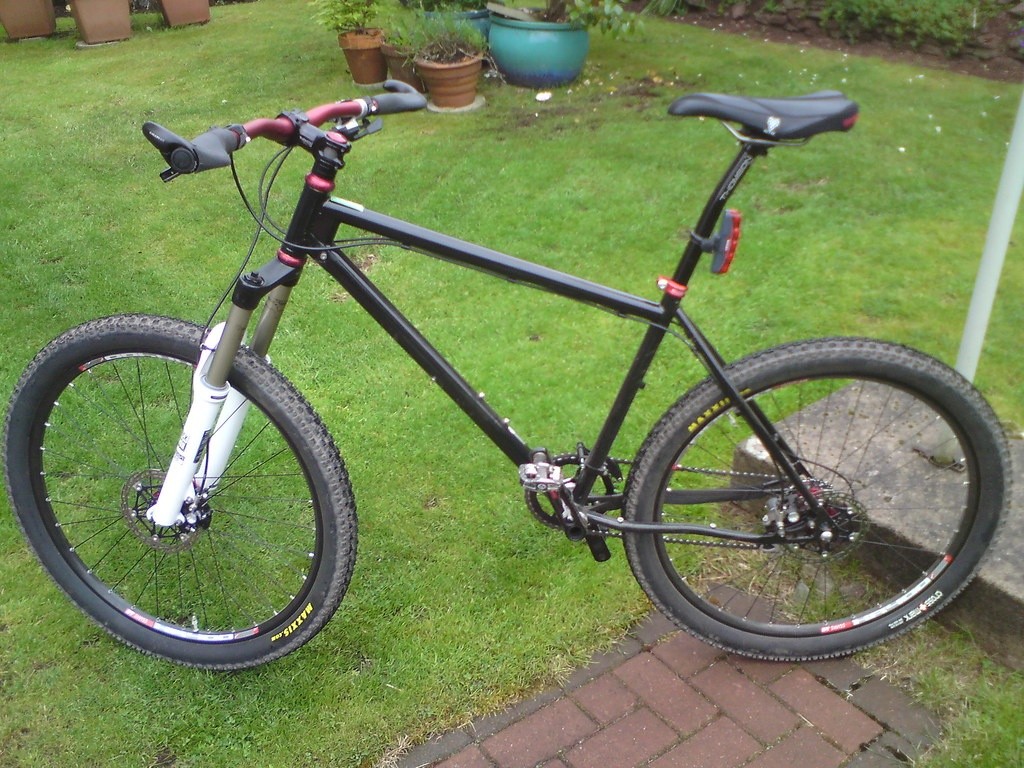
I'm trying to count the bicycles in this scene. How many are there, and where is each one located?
[3,79,1013,670]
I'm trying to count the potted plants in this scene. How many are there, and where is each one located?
[379,5,428,94]
[308,0,388,85]
[489,0,646,89]
[414,0,504,67]
[413,4,491,106]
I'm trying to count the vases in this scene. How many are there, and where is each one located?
[157,0,211,26]
[68,0,132,44]
[0,0,58,41]
